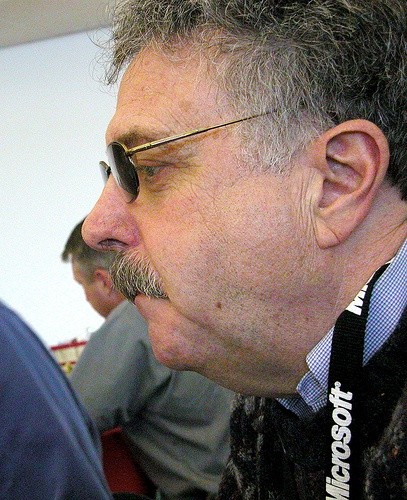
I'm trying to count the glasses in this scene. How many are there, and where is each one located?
[98,101,307,205]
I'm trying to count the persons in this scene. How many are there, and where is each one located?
[1,298,117,499]
[81,0,407,500]
[61,219,245,499]
